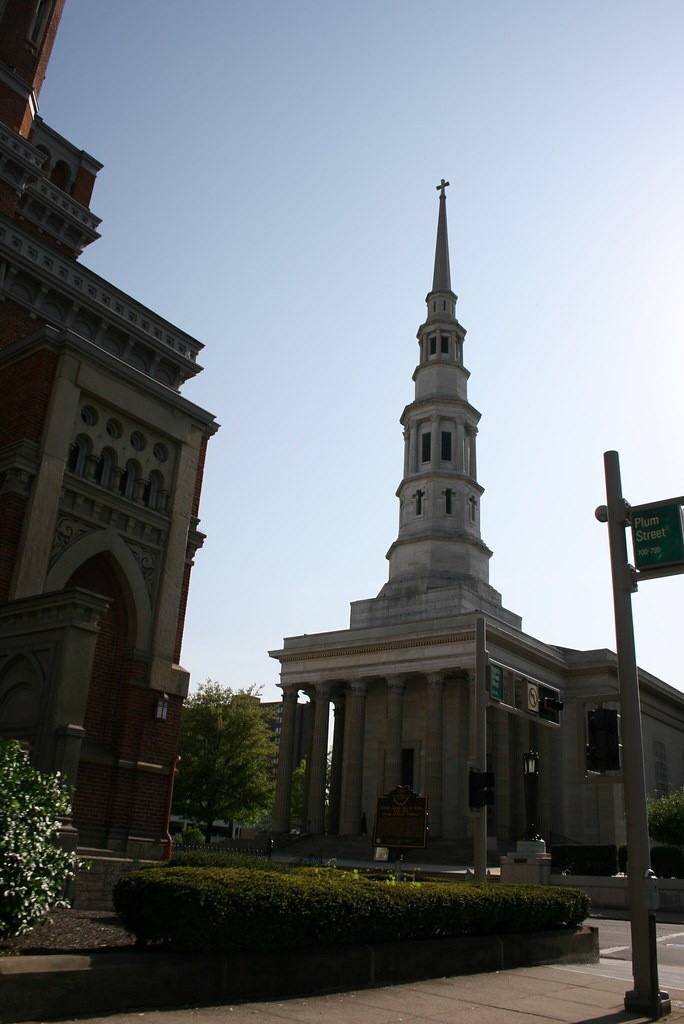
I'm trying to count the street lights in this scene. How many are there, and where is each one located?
[523,749,540,841]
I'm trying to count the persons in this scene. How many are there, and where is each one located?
[361,812,367,834]
